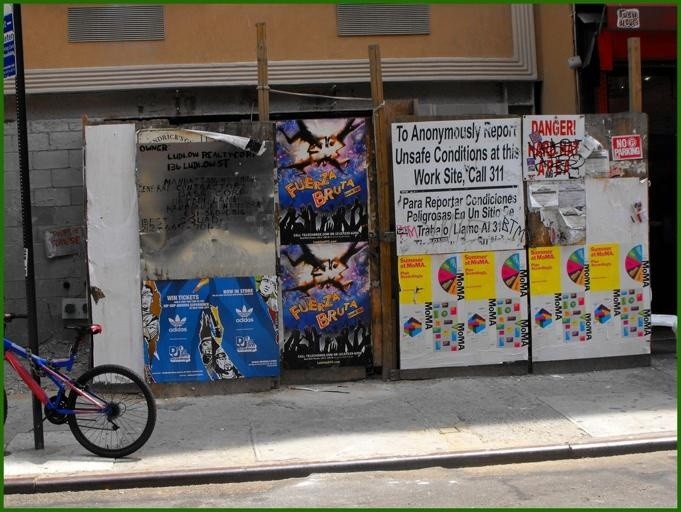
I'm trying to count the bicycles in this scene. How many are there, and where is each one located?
[2,313,157,458]
